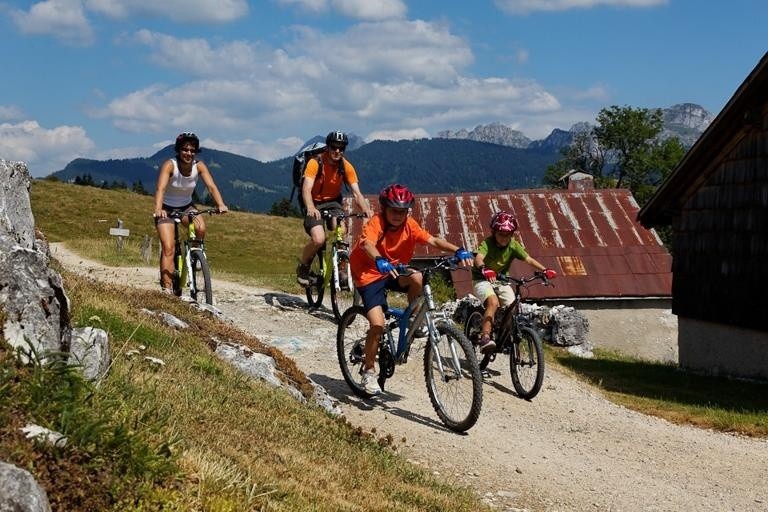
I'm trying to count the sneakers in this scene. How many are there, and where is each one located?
[409,322,430,338]
[193,244,205,272]
[361,371,382,395]
[296,264,311,287]
[338,270,348,287]
[481,334,497,354]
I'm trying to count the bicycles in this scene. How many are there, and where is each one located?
[304,209,366,326]
[152,209,221,305]
[466,269,555,399]
[338,255,482,431]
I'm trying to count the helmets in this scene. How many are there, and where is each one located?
[176,133,199,148]
[378,183,415,209]
[326,131,348,145]
[490,211,518,232]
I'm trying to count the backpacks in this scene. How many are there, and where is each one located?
[293,143,344,209]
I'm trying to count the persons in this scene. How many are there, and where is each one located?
[348,184,475,397]
[470,212,558,354]
[152,131,229,296]
[295,131,374,286]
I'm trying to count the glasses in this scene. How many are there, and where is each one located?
[330,145,346,152]
[181,148,197,153]
